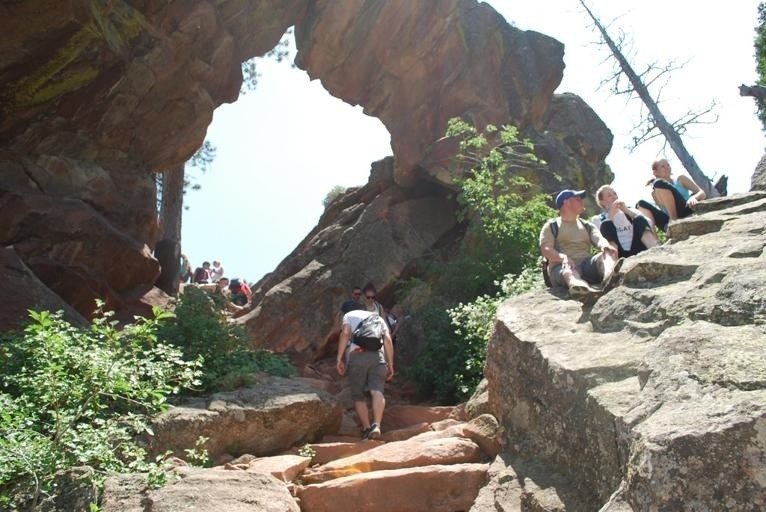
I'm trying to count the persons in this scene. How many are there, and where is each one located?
[177,255,255,310]
[636,158,706,245]
[382,306,401,384]
[592,186,660,257]
[335,301,394,440]
[341,287,364,312]
[359,282,393,334]
[537,188,624,305]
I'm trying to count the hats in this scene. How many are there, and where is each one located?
[553,188,587,209]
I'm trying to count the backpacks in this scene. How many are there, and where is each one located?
[348,311,386,352]
[538,217,559,288]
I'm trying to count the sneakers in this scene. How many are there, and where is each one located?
[359,422,384,441]
[600,255,626,294]
[568,284,605,305]
